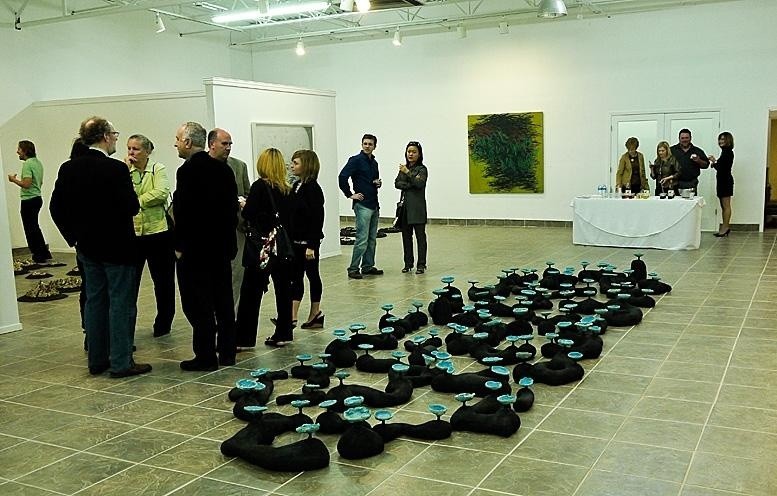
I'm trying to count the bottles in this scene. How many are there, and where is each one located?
[660,181,666,199]
[641,178,650,198]
[625,182,631,196]
[667,177,673,199]
[597,184,605,197]
[615,184,621,197]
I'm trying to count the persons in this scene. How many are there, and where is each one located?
[235,146,293,347]
[394,141,428,274]
[207,128,250,307]
[338,134,383,279]
[649,129,734,237]
[8,140,52,263]
[616,137,646,193]
[173,122,238,371]
[125,135,175,337]
[270,149,324,329]
[49,117,152,378]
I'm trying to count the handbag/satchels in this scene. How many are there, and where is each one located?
[392,190,408,229]
[242,227,295,266]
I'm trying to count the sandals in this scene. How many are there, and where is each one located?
[269,317,297,329]
[416,266,424,274]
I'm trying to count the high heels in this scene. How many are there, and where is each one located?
[713,228,730,237]
[301,310,324,329]
[402,268,411,273]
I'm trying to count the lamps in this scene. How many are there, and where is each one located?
[153,13,165,34]
[295,17,402,55]
[536,0,567,19]
[456,16,508,39]
[340,0,371,12]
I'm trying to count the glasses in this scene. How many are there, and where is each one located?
[111,131,119,137]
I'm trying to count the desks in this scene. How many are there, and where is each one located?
[570,196,705,251]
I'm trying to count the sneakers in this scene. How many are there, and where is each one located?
[180,357,218,371]
[348,271,362,279]
[362,268,383,275]
[111,363,152,378]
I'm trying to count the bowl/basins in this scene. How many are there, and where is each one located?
[677,188,694,198]
